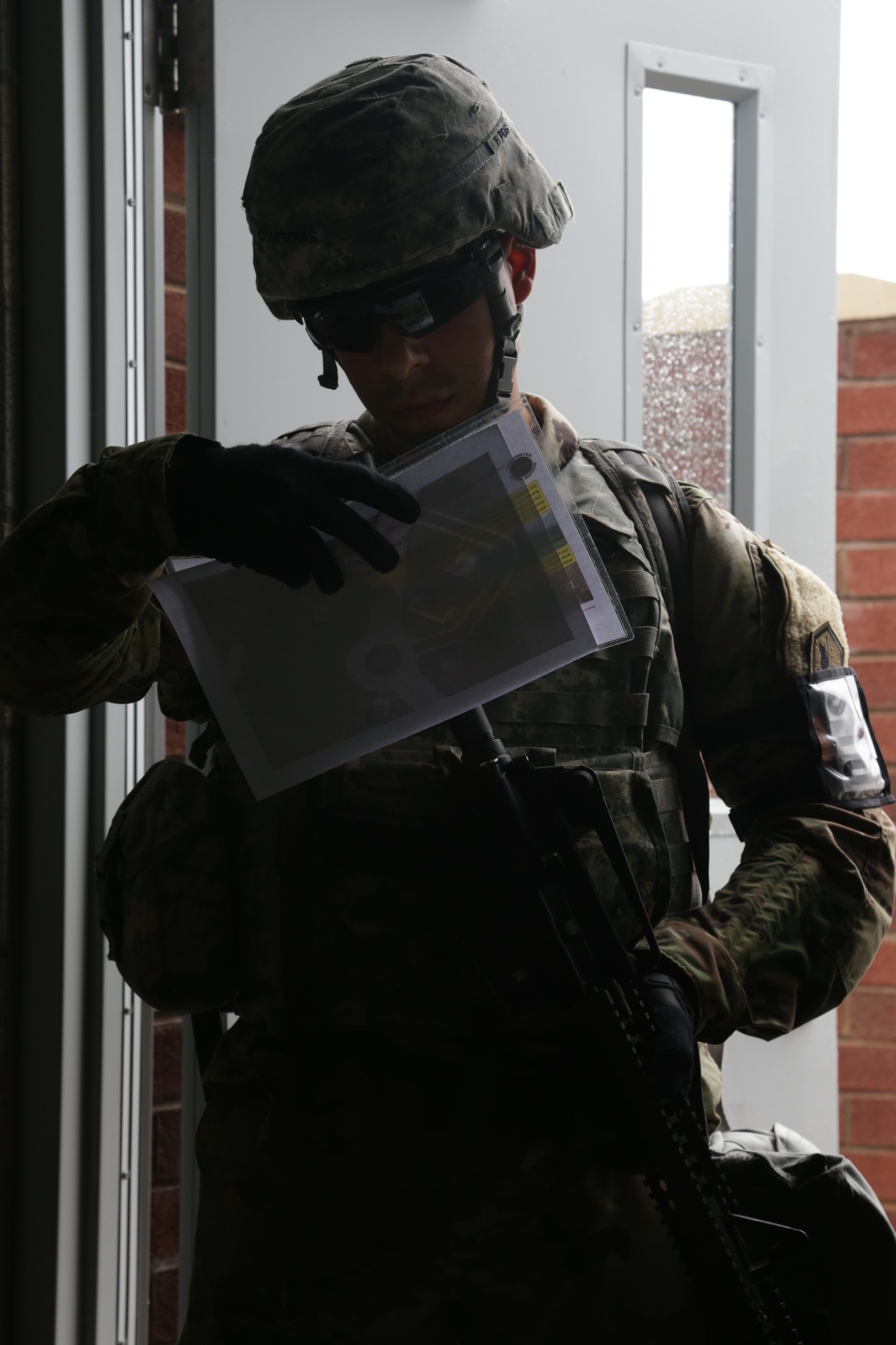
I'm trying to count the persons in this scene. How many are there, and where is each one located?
[0,47,896,1345]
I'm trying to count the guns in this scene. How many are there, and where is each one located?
[454,705,791,1342]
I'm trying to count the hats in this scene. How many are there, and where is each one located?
[241,53,573,308]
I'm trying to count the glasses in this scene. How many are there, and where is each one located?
[298,239,497,353]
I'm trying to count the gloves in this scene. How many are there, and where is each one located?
[172,441,424,596]
[634,971,699,1093]
